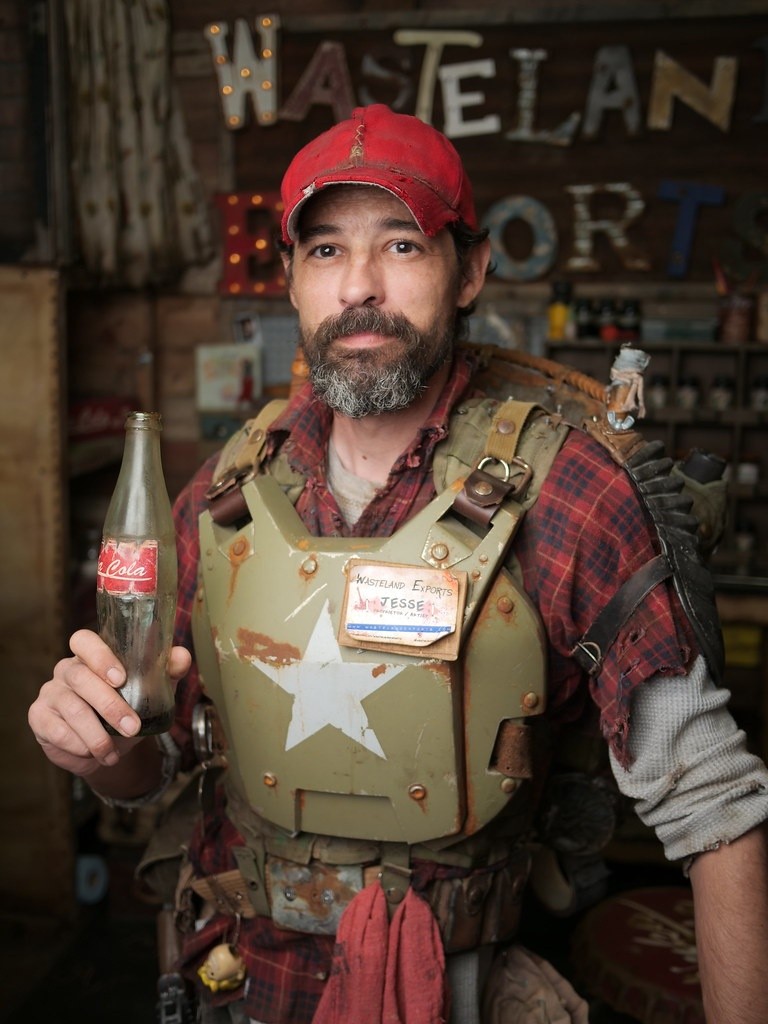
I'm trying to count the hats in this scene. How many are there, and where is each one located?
[280,103,479,244]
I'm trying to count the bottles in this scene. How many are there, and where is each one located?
[97,413,180,736]
[546,282,640,341]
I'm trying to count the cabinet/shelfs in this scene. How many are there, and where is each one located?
[0,264,166,928]
[532,333,767,591]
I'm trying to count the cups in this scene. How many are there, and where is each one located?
[709,377,733,409]
[652,377,671,408]
[751,377,768,411]
[678,377,699,410]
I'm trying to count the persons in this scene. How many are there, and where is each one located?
[29,105,768,1024]
